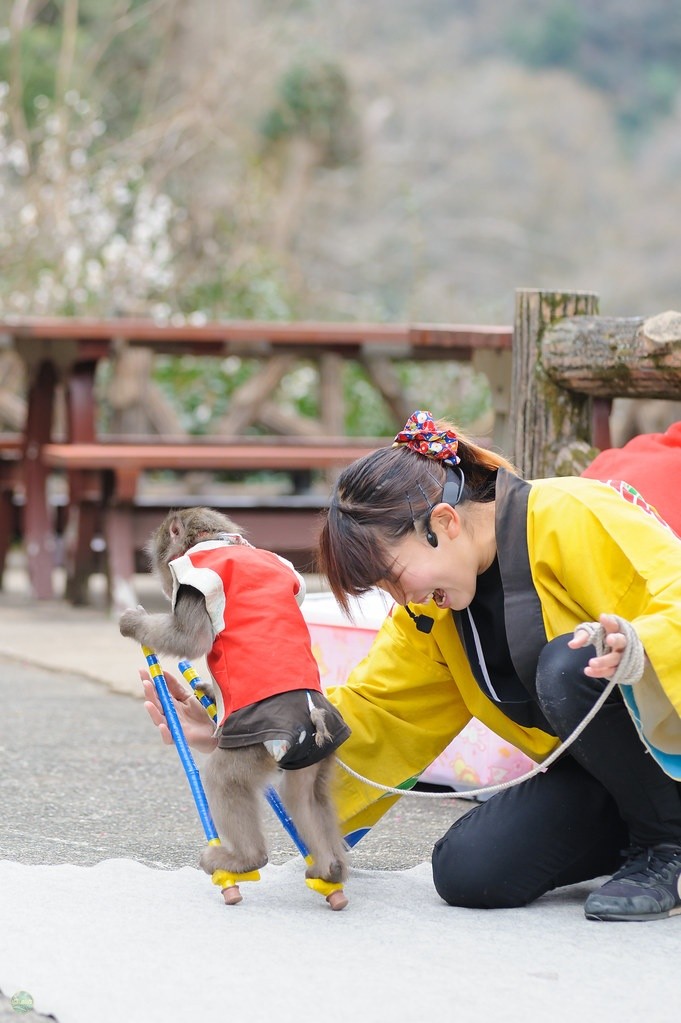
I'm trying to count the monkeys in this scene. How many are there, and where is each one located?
[118,506,349,884]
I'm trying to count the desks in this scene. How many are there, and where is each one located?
[1,316,614,609]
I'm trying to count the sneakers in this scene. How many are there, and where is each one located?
[585,843,681,923]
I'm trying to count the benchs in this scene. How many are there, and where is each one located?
[1,428,334,595]
[39,440,387,624]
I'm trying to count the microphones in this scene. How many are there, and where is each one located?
[404,605,434,633]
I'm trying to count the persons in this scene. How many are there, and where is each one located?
[139,412,680,922]
